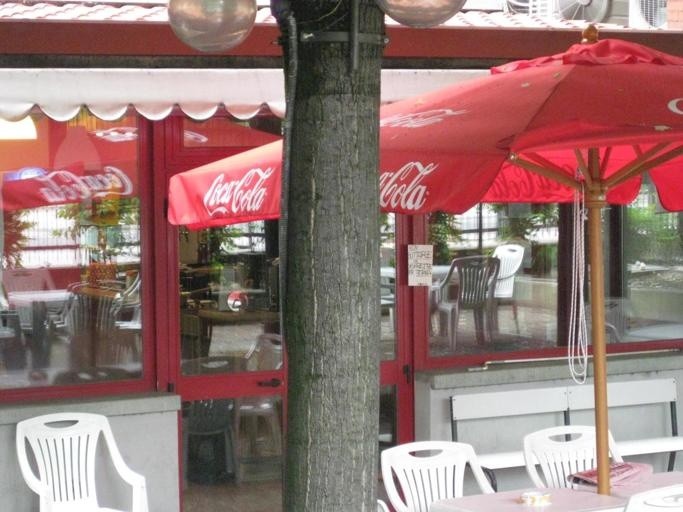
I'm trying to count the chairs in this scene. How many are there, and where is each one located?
[377,426,625,512]
[181,312,282,483]
[16,412,149,510]
[0,267,142,367]
[433,243,524,349]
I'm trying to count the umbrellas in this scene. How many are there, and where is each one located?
[168,24,683,496]
[0,104,283,212]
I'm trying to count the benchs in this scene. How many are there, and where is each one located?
[449,378,683,493]
